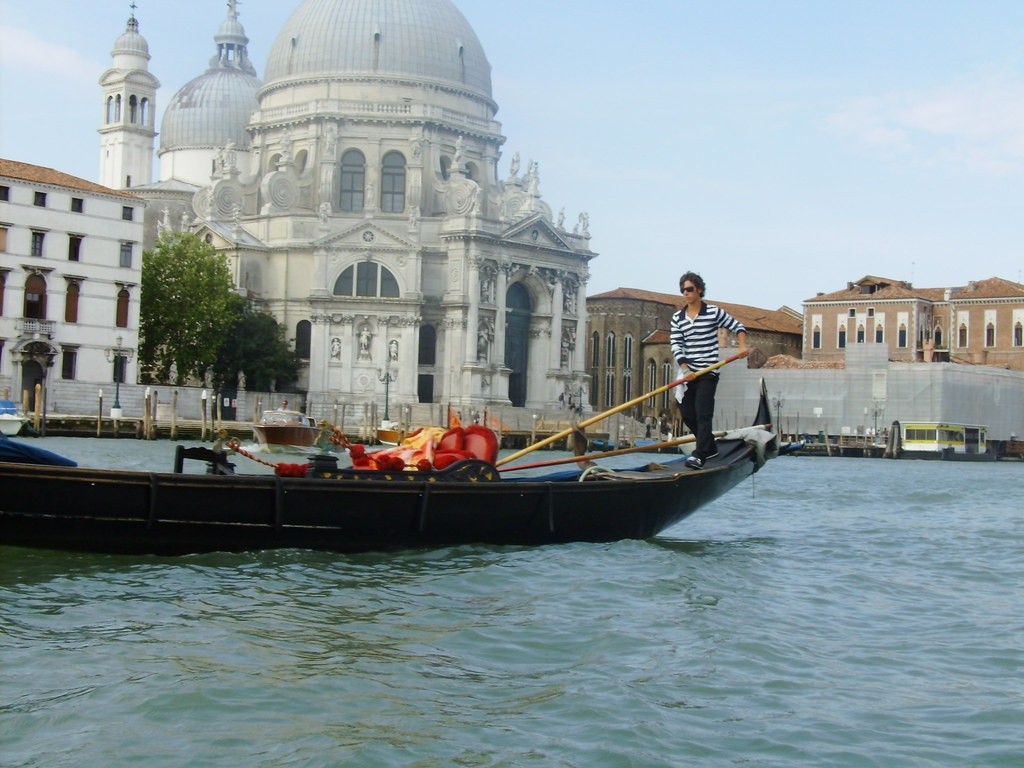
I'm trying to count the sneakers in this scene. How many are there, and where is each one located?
[685,451,706,471]
[705,450,719,460]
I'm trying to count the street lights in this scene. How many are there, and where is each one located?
[871,403,883,437]
[773,391,785,434]
[376,358,399,430]
[104,335,134,419]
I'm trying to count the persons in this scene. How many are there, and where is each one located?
[524,158,541,193]
[365,182,374,206]
[157,207,189,245]
[481,278,490,302]
[456,411,462,421]
[562,338,569,361]
[453,135,466,165]
[788,435,791,442]
[359,326,372,349]
[564,290,571,313]
[389,341,398,359]
[212,138,237,173]
[474,411,480,426]
[557,206,566,228]
[568,393,571,407]
[510,150,520,178]
[331,339,341,360]
[277,128,292,159]
[408,130,429,163]
[477,329,488,358]
[276,400,291,422]
[408,206,417,228]
[559,392,564,409]
[573,212,590,233]
[320,203,329,224]
[670,272,750,470]
[320,126,340,160]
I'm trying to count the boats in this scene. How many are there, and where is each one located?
[252,400,323,446]
[1,399,803,548]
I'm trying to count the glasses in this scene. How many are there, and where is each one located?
[680,286,694,294]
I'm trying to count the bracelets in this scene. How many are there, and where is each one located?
[683,368,690,375]
